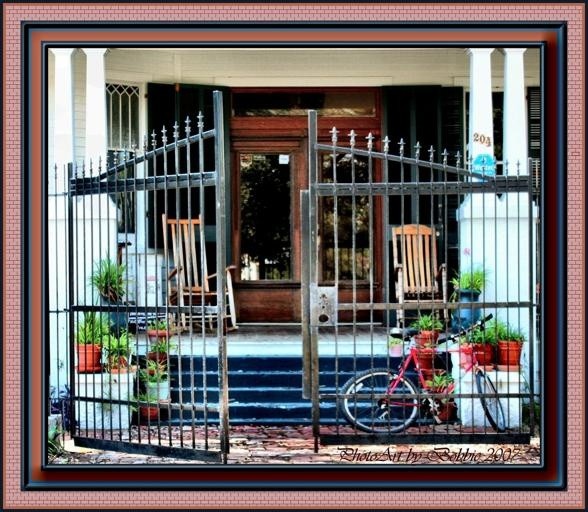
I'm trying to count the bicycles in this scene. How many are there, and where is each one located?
[338,313,506,434]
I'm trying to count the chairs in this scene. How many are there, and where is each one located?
[388,225,451,336]
[161,213,240,334]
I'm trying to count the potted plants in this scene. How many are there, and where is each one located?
[130,392,163,420]
[407,313,454,425]
[89,256,130,332]
[74,302,103,375]
[462,326,496,371]
[497,322,524,371]
[101,330,139,373]
[140,367,172,402]
[145,320,173,345]
[146,344,169,374]
[449,269,488,335]
